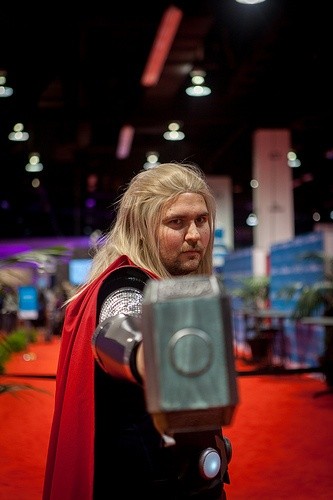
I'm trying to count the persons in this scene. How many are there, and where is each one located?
[2,279,73,338]
[42,163,240,500]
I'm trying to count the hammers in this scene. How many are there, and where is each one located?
[145,275,238,433]
